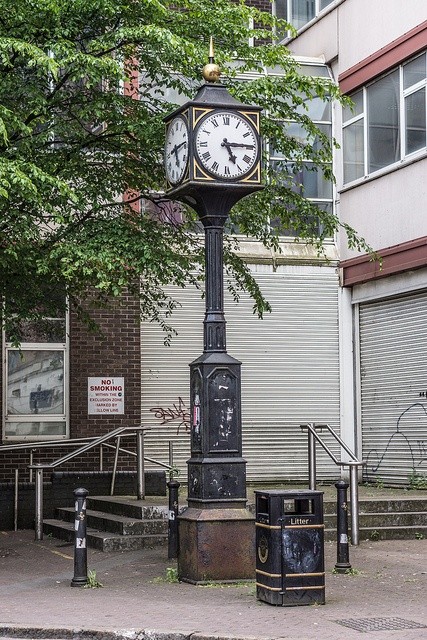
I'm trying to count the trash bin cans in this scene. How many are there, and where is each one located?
[253,489,325,606]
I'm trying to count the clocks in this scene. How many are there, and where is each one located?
[192,107,261,185]
[163,114,190,188]
